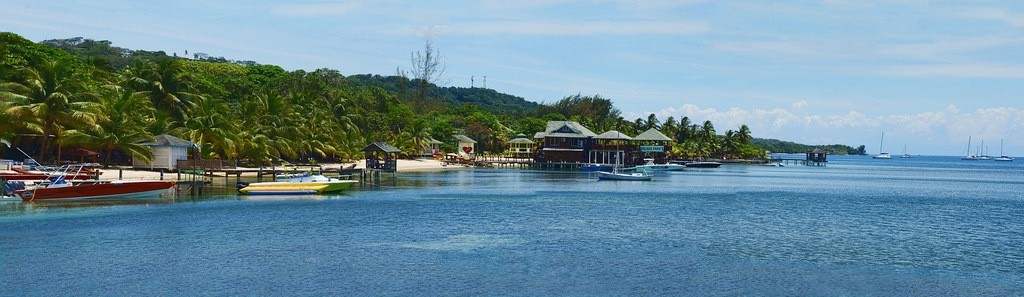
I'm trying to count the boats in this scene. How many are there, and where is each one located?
[686,160,721,168]
[871,132,891,158]
[634,161,684,170]
[15,178,175,203]
[765,162,785,167]
[581,164,615,172]
[598,130,654,180]
[236,172,359,194]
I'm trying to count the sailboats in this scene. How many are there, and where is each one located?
[996,139,1014,160]
[900,144,912,158]
[961,135,978,160]
[976,139,993,160]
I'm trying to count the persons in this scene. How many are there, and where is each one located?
[370,157,374,168]
[346,164,357,169]
[697,156,701,162]
